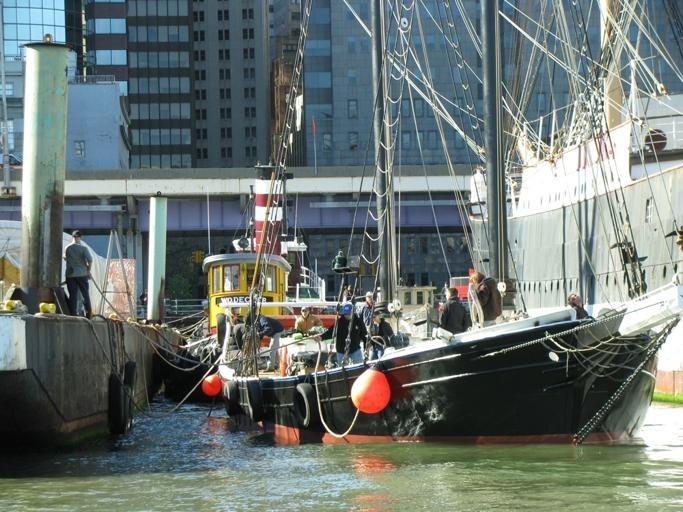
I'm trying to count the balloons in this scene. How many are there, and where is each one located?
[353,455,395,505]
[349,368,393,414]
[201,374,222,399]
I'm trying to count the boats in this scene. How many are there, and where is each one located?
[202,161,366,335]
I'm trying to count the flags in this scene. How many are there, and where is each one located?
[310,119,318,136]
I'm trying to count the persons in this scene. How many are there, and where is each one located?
[567,291,590,319]
[63,228,93,319]
[231,271,504,373]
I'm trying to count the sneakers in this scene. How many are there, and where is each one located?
[263,368,274,372]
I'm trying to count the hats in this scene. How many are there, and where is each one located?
[341,304,351,315]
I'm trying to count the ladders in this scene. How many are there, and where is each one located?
[99,229,135,321]
